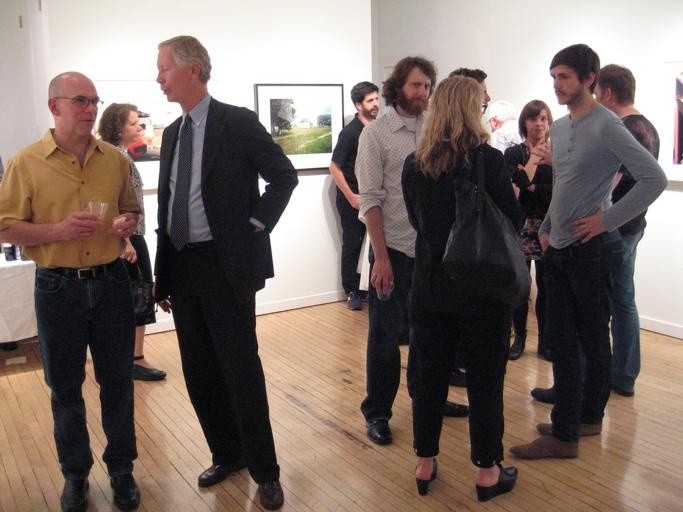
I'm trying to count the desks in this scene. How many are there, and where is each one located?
[0,252,40,347]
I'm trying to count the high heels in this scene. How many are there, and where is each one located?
[414,456,436,496]
[474,461,517,502]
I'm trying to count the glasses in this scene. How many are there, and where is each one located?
[51,95,99,109]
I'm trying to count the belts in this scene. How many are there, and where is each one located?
[184,240,212,249]
[35,257,125,280]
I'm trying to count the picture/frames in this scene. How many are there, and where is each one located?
[254,83,344,172]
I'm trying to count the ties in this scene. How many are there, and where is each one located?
[170,114,192,253]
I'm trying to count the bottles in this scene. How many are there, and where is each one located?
[2,243,17,261]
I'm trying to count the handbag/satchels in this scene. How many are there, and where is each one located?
[515,206,545,262]
[128,276,156,327]
[440,175,532,312]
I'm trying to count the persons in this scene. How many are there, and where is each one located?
[592,62,661,399]
[96,102,167,383]
[0,71,145,512]
[401,73,529,502]
[149,34,300,511]
[329,80,382,311]
[436,67,506,150]
[508,44,668,461]
[502,100,554,362]
[353,55,471,447]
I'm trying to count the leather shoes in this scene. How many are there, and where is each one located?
[530,387,555,405]
[59,477,90,511]
[257,479,284,511]
[345,289,362,311]
[365,418,393,445]
[610,383,634,397]
[107,472,140,511]
[535,422,602,436]
[442,399,469,418]
[197,459,243,488]
[446,365,467,387]
[131,362,166,381]
[537,343,553,361]
[508,433,579,459]
[507,333,525,360]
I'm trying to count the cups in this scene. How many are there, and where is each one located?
[89,201,108,221]
